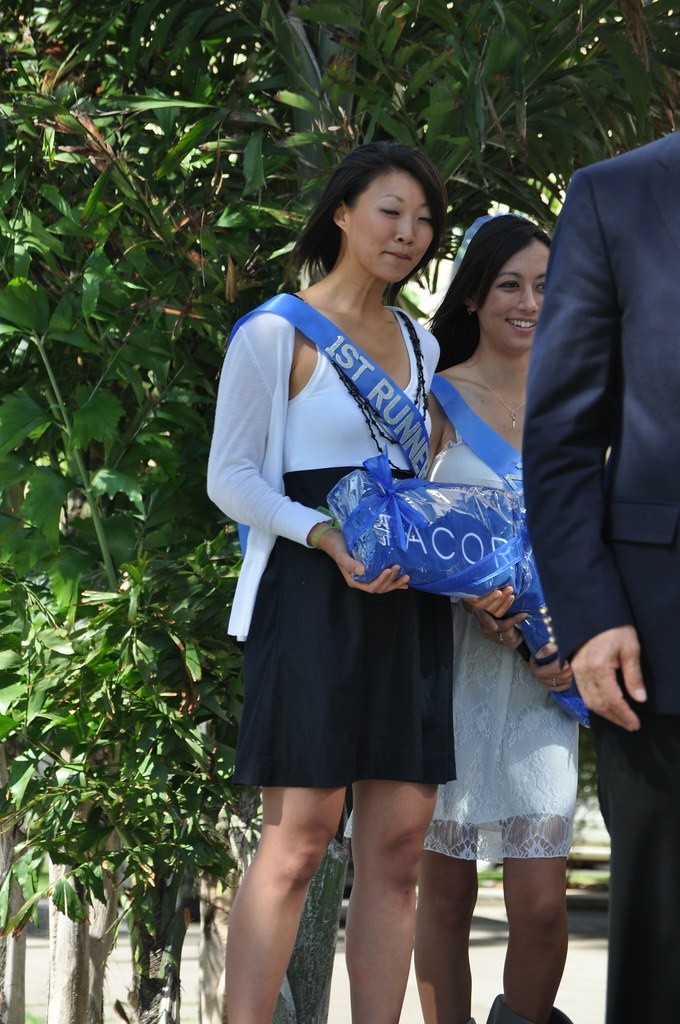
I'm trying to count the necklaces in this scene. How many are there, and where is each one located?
[467,356,527,429]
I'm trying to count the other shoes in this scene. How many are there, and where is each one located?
[486,994,574,1024]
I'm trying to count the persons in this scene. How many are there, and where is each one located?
[414,213,616,1024]
[514,121,680,1024]
[204,149,518,1024]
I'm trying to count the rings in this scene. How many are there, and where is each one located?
[552,678,557,687]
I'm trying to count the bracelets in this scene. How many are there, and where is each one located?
[311,505,341,548]
